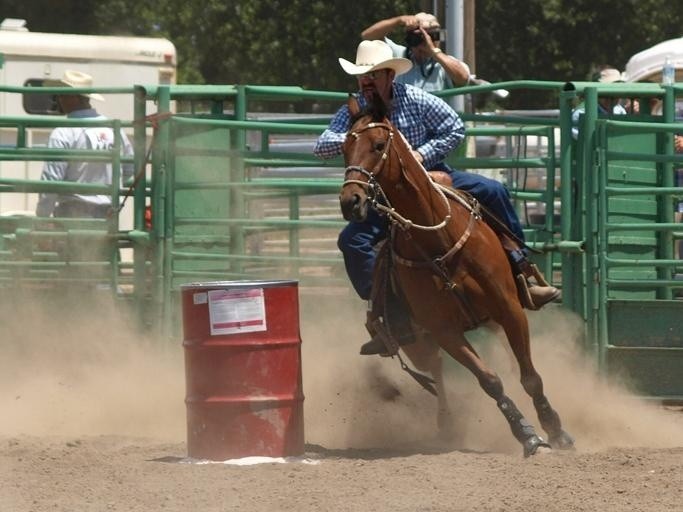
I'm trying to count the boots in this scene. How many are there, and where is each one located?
[360,293,416,355]
[518,270,561,306]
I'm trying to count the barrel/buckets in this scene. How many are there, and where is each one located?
[179,279,306,459]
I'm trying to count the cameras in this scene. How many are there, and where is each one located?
[405,27,447,47]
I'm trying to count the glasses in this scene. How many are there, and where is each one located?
[355,72,389,80]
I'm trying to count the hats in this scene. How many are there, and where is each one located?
[338,40,413,78]
[598,69,625,84]
[41,69,105,103]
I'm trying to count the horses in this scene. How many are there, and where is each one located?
[339,83,575,460]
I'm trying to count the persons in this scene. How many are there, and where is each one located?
[571,68,683,260]
[361,12,470,100]
[36,71,135,279]
[313,39,560,354]
